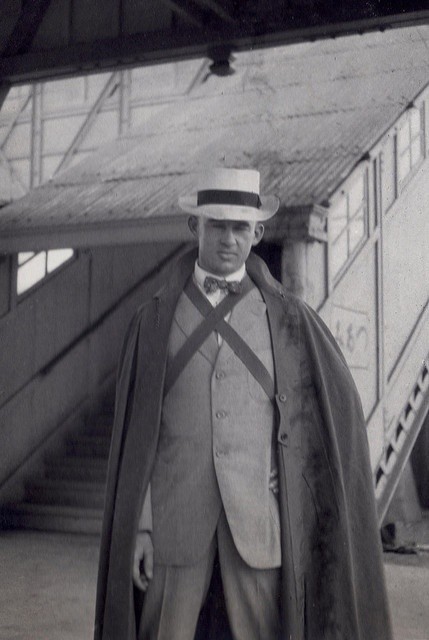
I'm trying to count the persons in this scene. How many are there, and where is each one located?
[96,167,395,640]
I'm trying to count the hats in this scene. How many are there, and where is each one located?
[174,166,281,222]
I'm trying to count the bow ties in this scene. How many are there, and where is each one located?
[202,275,243,295]
